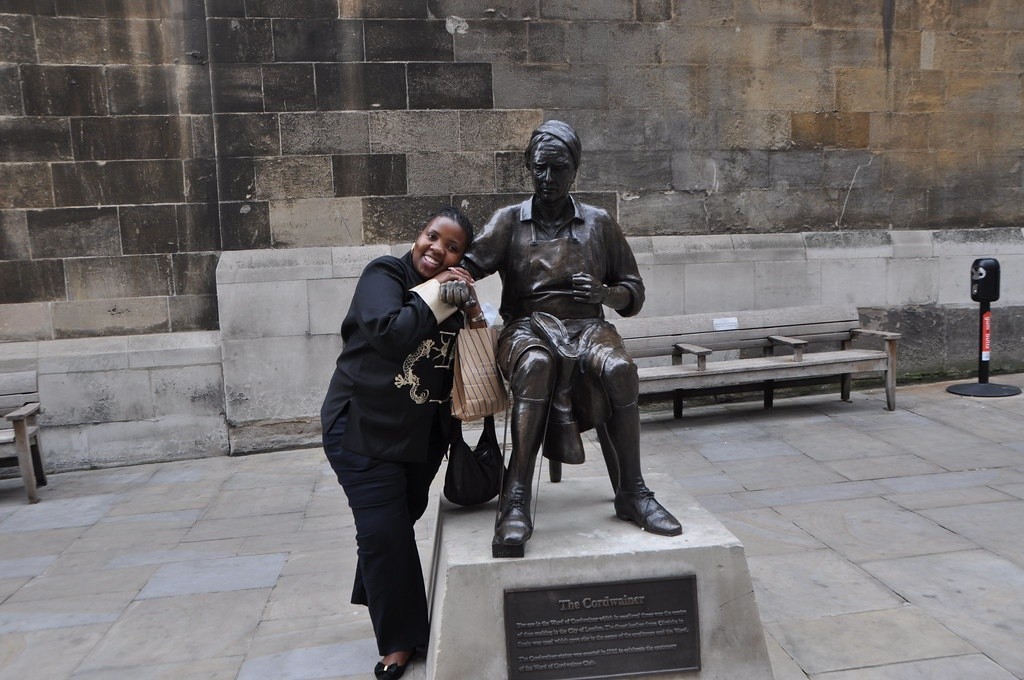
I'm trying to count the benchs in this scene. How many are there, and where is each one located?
[0,371,47,504]
[598,303,901,418]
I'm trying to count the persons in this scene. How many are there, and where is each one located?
[320,207,488,678]
[439,121,682,557]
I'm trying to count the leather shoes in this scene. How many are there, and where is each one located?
[375,654,412,680]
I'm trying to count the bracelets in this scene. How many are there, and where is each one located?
[468,311,484,324]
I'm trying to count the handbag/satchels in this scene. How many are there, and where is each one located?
[443,327,507,509]
